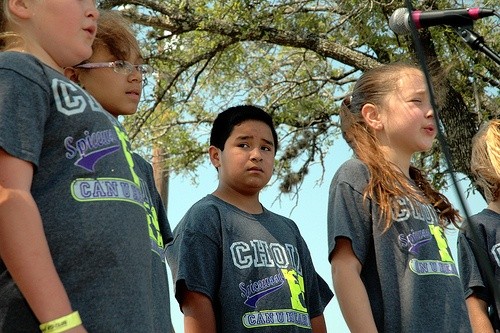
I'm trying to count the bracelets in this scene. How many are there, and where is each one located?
[39,310,82,333]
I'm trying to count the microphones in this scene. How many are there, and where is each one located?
[389,7,495,34]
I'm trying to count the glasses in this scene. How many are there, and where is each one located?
[76,59,146,76]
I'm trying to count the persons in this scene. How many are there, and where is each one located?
[164,105,334,332]
[457,118,500,333]
[0,0,154,333]
[62,10,176,332]
[328,65,472,333]
